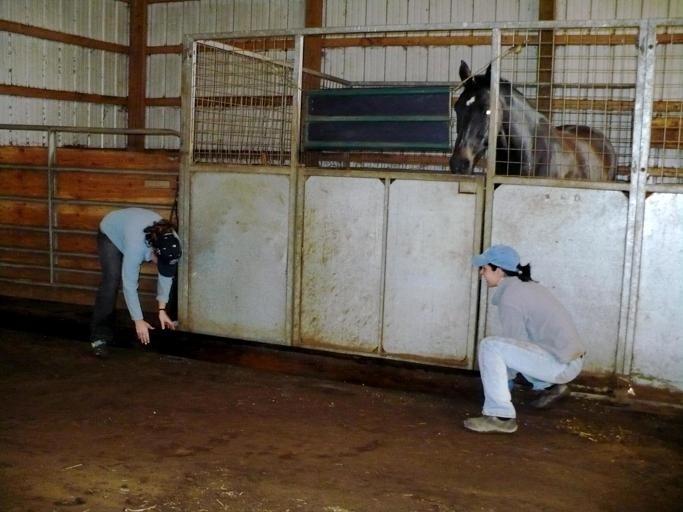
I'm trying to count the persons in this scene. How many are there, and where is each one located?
[463,243,587,432]
[82,206,183,359]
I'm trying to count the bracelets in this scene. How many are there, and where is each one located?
[158,308,165,310]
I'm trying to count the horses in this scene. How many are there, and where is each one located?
[448,60,618,182]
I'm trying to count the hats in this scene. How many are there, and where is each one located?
[471,244,521,273]
[157,233,182,276]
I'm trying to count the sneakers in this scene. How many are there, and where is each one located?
[93,339,110,359]
[530,383,571,409]
[464,415,518,434]
